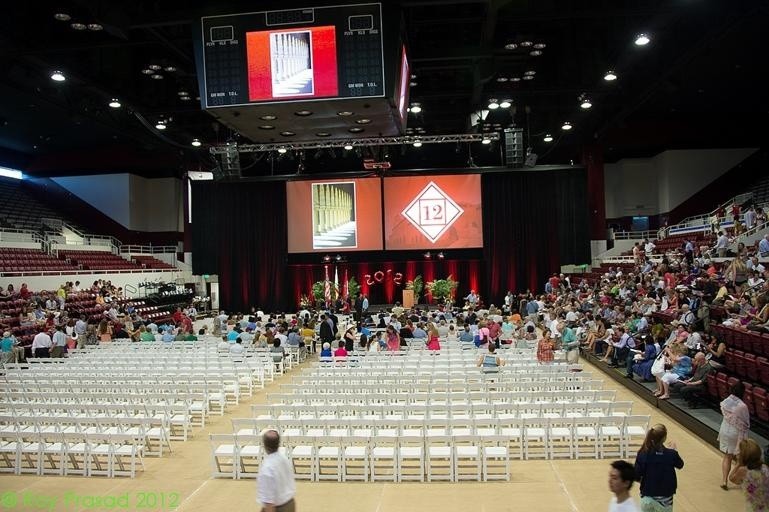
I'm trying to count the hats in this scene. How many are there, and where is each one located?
[346,324,354,331]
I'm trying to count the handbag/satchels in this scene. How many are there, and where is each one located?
[480,335,487,344]
[651,358,664,375]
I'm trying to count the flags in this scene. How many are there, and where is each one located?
[325,266,349,311]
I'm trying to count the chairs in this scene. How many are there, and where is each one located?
[567,232,768,421]
[1,248,183,349]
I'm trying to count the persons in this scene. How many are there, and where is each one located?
[607,458,641,512]
[631,422,685,512]
[1,281,96,372]
[592,200,769,411]
[728,437,769,511]
[92,280,338,352]
[716,381,750,490]
[255,429,296,512]
[322,273,598,373]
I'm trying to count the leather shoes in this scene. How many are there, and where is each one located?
[584,341,595,354]
[653,388,669,400]
[624,373,633,378]
[598,358,618,369]
[720,482,728,488]
[639,378,647,383]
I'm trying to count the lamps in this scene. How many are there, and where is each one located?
[48,66,66,82]
[578,92,593,109]
[561,119,573,130]
[155,120,166,130]
[192,137,202,147]
[109,97,121,108]
[544,130,554,142]
[602,69,620,81]
[632,31,652,46]
